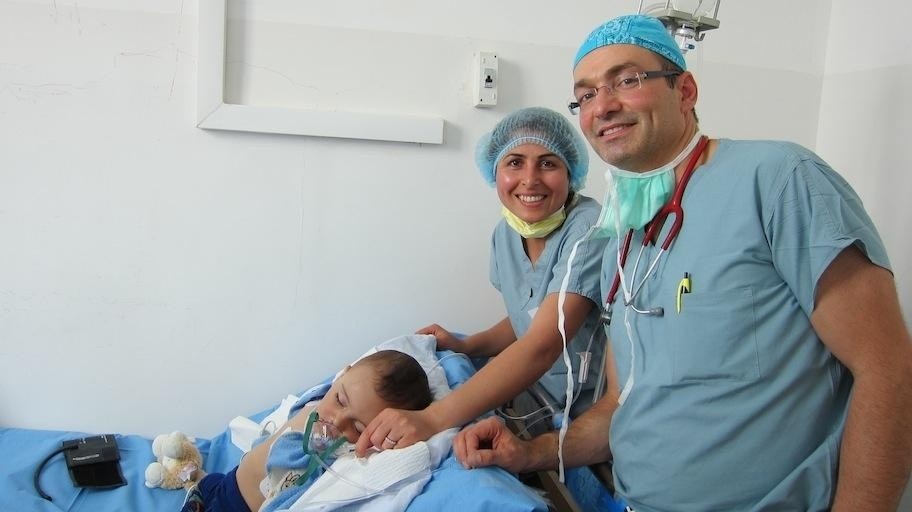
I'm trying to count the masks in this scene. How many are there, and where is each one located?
[499,207,567,240]
[583,167,676,239]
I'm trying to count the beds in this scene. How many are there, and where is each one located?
[0,330,634,510]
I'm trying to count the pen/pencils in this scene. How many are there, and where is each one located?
[677,272,690,314]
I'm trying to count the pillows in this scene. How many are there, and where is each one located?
[290,334,462,509]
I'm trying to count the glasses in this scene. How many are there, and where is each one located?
[568,68,680,115]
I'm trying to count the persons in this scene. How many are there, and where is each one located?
[354,105,614,494]
[448,13,911,511]
[179,349,437,512]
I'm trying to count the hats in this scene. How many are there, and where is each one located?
[573,14,686,71]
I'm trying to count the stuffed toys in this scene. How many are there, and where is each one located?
[144,429,209,492]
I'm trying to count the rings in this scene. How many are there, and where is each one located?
[384,436,397,445]
